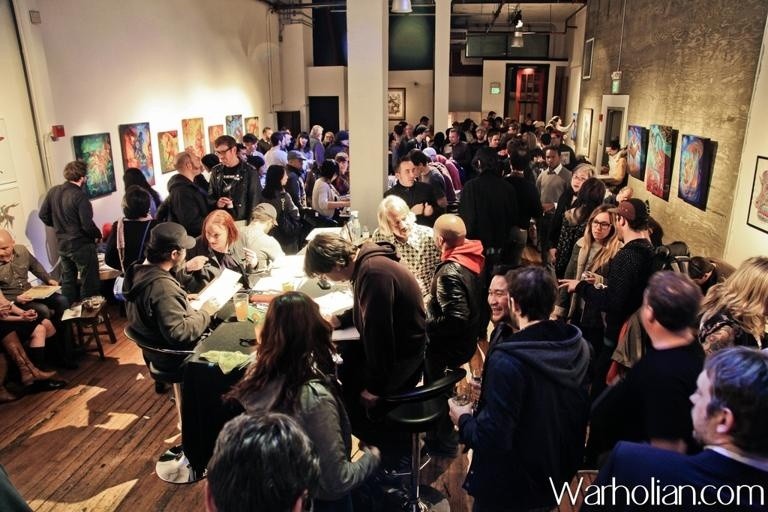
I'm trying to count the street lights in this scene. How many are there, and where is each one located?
[113,274,127,300]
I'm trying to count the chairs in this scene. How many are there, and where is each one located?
[0,167,487,511]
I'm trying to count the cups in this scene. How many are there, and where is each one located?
[215,147,233,155]
[591,220,610,230]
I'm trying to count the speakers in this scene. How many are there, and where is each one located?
[1,331,78,402]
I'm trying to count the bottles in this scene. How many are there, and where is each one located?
[608,198,646,221]
[288,151,307,160]
[423,148,437,161]
[254,203,279,227]
[151,223,197,252]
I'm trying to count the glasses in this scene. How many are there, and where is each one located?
[391,1,413,15]
[510,10,524,49]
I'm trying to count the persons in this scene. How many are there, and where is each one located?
[0,111,768,511]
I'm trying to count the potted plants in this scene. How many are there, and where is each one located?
[387,87,407,121]
[745,155,768,235]
[579,107,594,158]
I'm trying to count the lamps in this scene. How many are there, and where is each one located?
[391,1,413,15]
[510,10,524,49]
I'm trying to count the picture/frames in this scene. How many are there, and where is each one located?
[387,87,407,121]
[579,107,594,158]
[745,155,768,235]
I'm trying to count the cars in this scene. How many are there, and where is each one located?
[471,365,483,383]
[226,198,235,208]
[453,384,471,406]
[233,292,249,322]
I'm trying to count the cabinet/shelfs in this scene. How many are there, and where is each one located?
[361,226,371,239]
